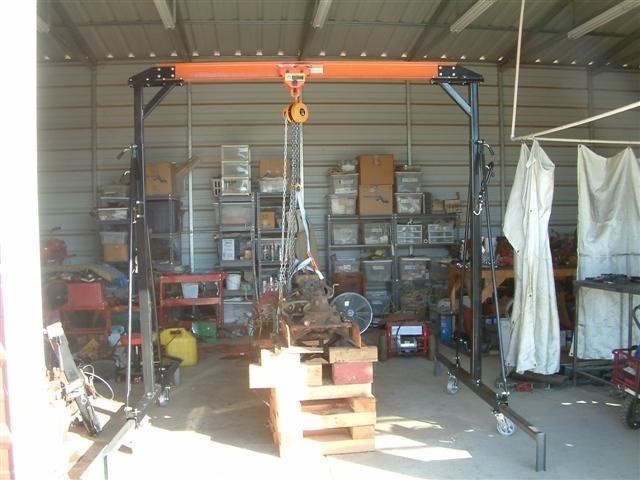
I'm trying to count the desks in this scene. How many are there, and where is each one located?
[445,267,576,350]
[569,278,640,403]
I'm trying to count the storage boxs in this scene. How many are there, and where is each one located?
[90,155,198,268]
[209,143,295,333]
[323,151,463,331]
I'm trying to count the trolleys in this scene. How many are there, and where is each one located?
[612,305,640,431]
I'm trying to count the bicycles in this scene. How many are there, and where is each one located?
[269,271,374,338]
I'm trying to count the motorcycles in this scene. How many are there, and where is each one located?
[40,226,100,436]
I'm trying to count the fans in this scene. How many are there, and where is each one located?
[328,292,374,335]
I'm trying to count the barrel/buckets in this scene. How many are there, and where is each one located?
[225,273,241,291]
[160,327,199,366]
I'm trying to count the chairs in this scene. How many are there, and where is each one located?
[57,282,112,346]
